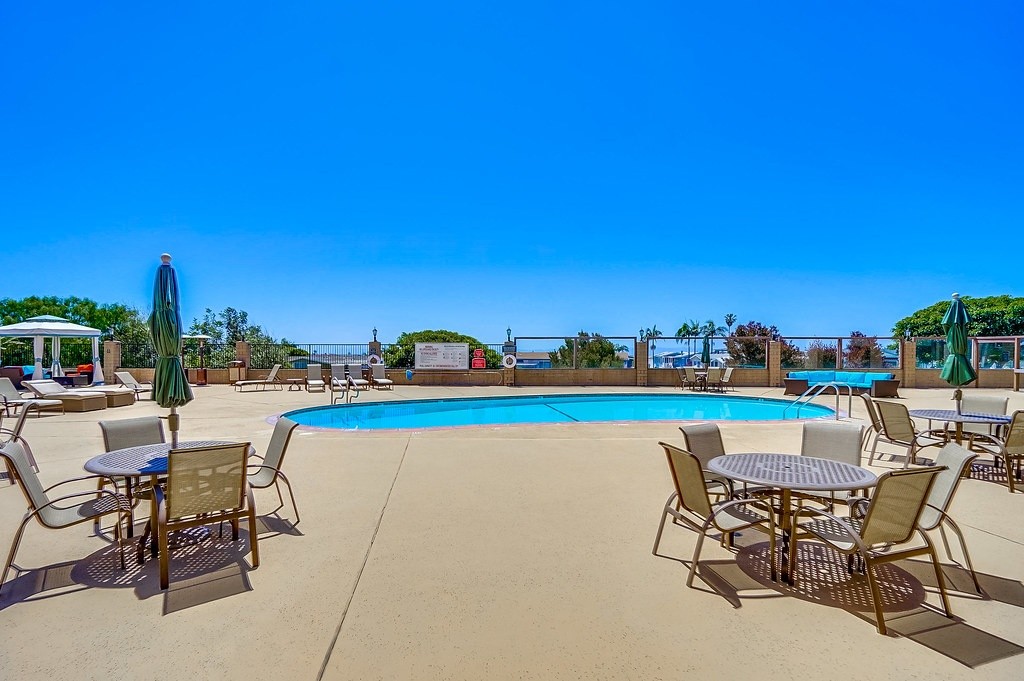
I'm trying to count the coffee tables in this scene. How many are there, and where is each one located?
[51,373,87,387]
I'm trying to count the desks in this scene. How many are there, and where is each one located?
[907,409,1011,479]
[287,378,303,391]
[706,452,877,583]
[83,441,256,565]
[684,372,722,391]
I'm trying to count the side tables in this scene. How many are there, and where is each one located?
[783,378,812,395]
[871,379,900,397]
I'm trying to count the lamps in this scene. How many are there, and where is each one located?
[905,327,911,341]
[109,328,114,341]
[240,329,246,341]
[506,326,512,341]
[639,327,644,341]
[372,326,377,342]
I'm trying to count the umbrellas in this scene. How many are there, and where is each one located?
[701,335,711,391]
[147,253,195,521]
[939,293,978,447]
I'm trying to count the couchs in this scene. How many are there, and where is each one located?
[785,370,896,397]
[0,366,52,390]
[65,364,94,385]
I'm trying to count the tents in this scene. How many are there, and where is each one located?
[0,315,104,386]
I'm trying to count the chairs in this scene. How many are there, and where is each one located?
[651,441,776,588]
[305,364,326,392]
[955,395,1011,449]
[787,465,950,635]
[0,378,301,590]
[234,364,284,393]
[704,367,724,393]
[867,400,952,469]
[792,420,867,521]
[672,423,773,550]
[846,442,990,595]
[965,409,1024,494]
[682,366,703,392]
[860,393,921,458]
[114,371,155,400]
[347,364,369,390]
[329,364,350,390]
[675,367,693,390]
[368,364,394,391]
[715,368,735,392]
